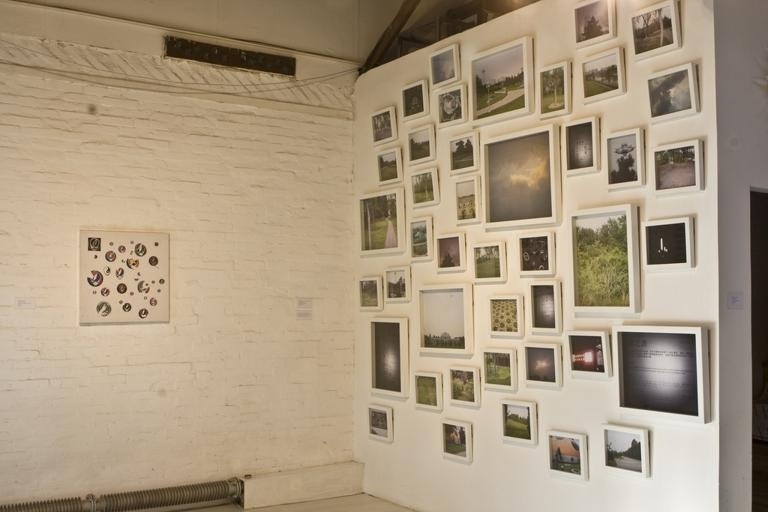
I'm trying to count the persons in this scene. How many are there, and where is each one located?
[383,208,397,249]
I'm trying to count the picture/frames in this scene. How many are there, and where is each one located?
[77,229,172,325]
[356,0,711,482]
